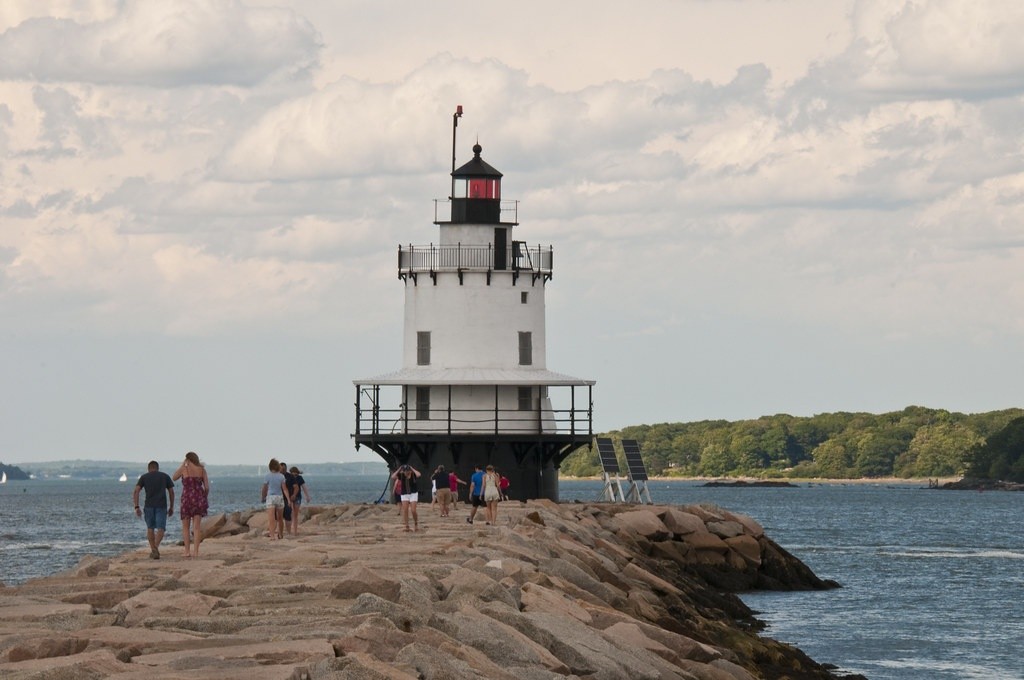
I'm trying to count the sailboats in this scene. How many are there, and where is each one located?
[118,473,127,482]
[138,475,141,479]
[0,472,7,485]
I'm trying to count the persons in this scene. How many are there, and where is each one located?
[261,459,310,540]
[133,461,175,559]
[389,464,510,532]
[173,452,209,557]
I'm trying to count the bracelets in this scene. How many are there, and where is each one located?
[135,506,139,509]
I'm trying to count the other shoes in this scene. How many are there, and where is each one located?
[404,523,419,532]
[267,534,284,539]
[441,514,449,517]
[467,517,474,524]
[150,548,160,559]
[485,522,490,525]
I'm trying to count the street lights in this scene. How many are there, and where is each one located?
[451,104,463,200]
